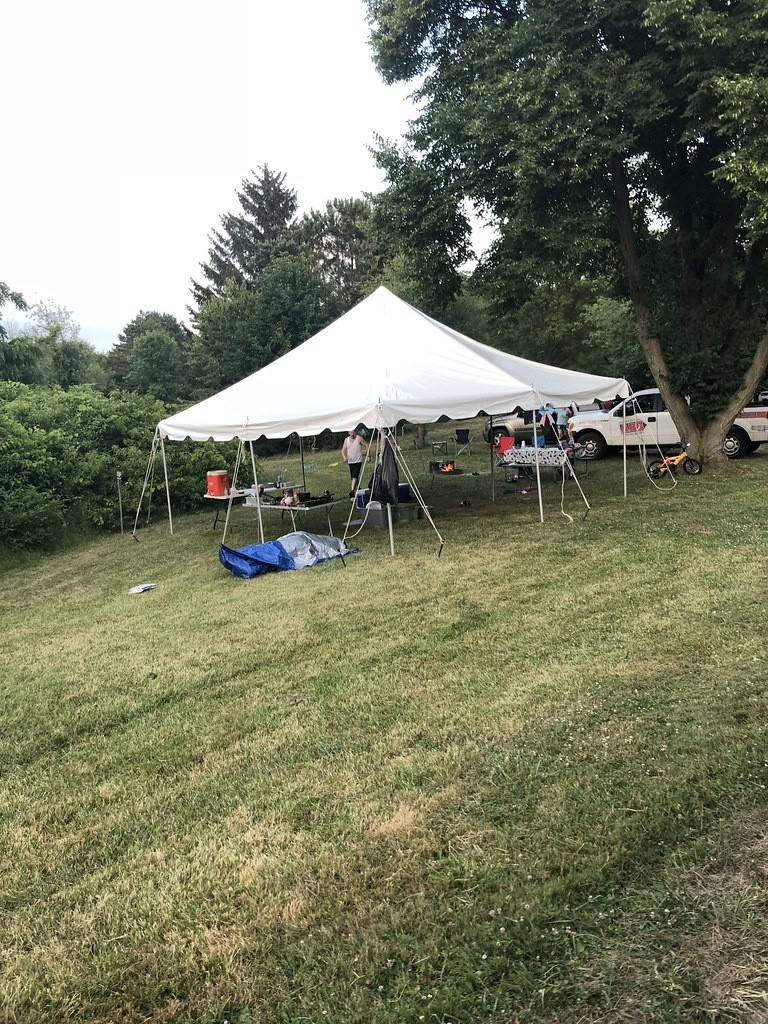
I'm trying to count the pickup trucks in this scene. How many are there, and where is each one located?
[566,387,768,460]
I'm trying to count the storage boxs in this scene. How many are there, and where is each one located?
[207,470,230,496]
[356,483,419,527]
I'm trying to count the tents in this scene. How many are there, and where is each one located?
[158,285,629,557]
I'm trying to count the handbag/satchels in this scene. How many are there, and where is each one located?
[367,438,400,506]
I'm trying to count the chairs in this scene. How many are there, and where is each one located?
[494,437,515,467]
[450,429,473,458]
[531,435,545,447]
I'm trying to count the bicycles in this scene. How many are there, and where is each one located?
[646,437,702,480]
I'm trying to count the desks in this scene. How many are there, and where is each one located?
[503,447,566,480]
[204,484,304,535]
[242,501,343,543]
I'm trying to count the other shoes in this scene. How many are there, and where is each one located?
[348,492,355,499]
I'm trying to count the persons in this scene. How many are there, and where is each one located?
[555,407,572,438]
[340,430,371,498]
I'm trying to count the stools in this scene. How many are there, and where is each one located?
[431,441,447,456]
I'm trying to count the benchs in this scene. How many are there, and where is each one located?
[499,461,561,483]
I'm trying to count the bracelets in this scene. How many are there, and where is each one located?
[368,456,371,457]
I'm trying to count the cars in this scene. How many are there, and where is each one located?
[758,390,768,402]
[481,399,632,446]
